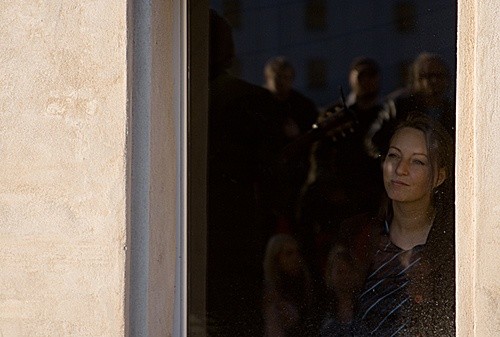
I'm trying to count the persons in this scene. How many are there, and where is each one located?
[206,50,456,337]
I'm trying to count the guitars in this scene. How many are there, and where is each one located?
[274,103,359,163]
